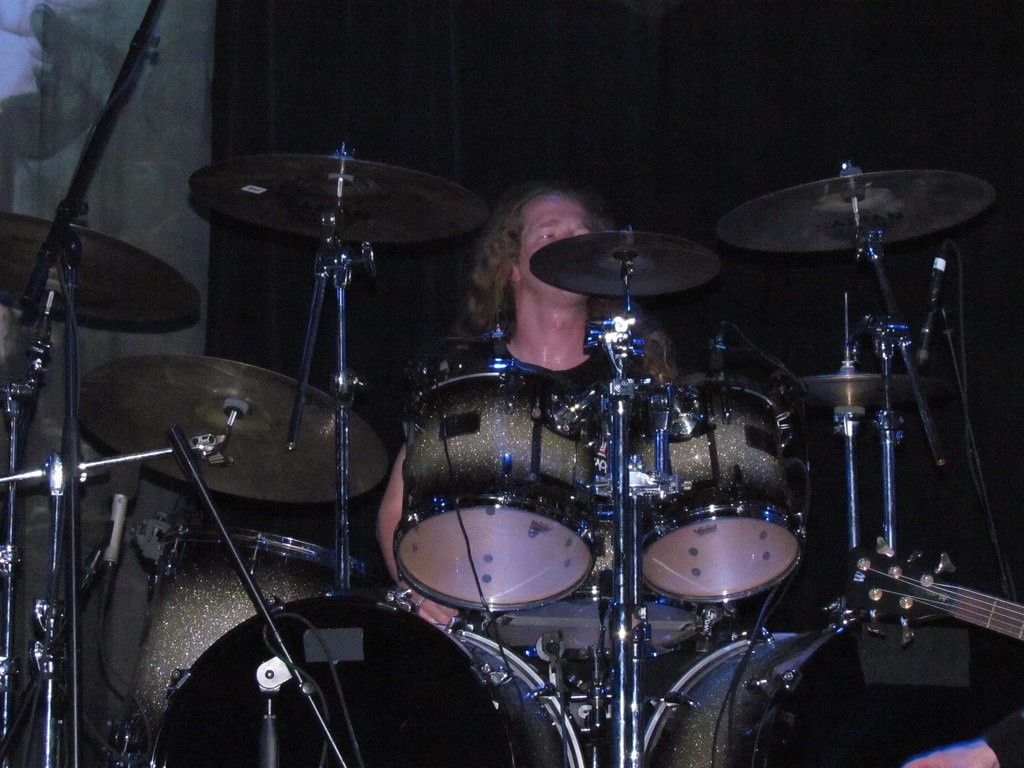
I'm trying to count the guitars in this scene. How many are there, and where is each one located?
[826,533,1024,648]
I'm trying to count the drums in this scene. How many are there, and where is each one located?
[391,357,599,614]
[643,609,1024,768]
[110,526,377,768]
[482,503,725,662]
[149,595,585,768]
[627,371,808,605]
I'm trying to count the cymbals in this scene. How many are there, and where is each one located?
[529,230,722,297]
[786,373,911,403]
[0,211,200,322]
[188,154,488,243]
[716,169,996,253]
[79,352,389,501]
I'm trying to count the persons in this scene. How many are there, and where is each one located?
[376,181,802,767]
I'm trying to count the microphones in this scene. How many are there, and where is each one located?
[916,239,951,366]
[710,321,728,371]
[98,461,141,605]
